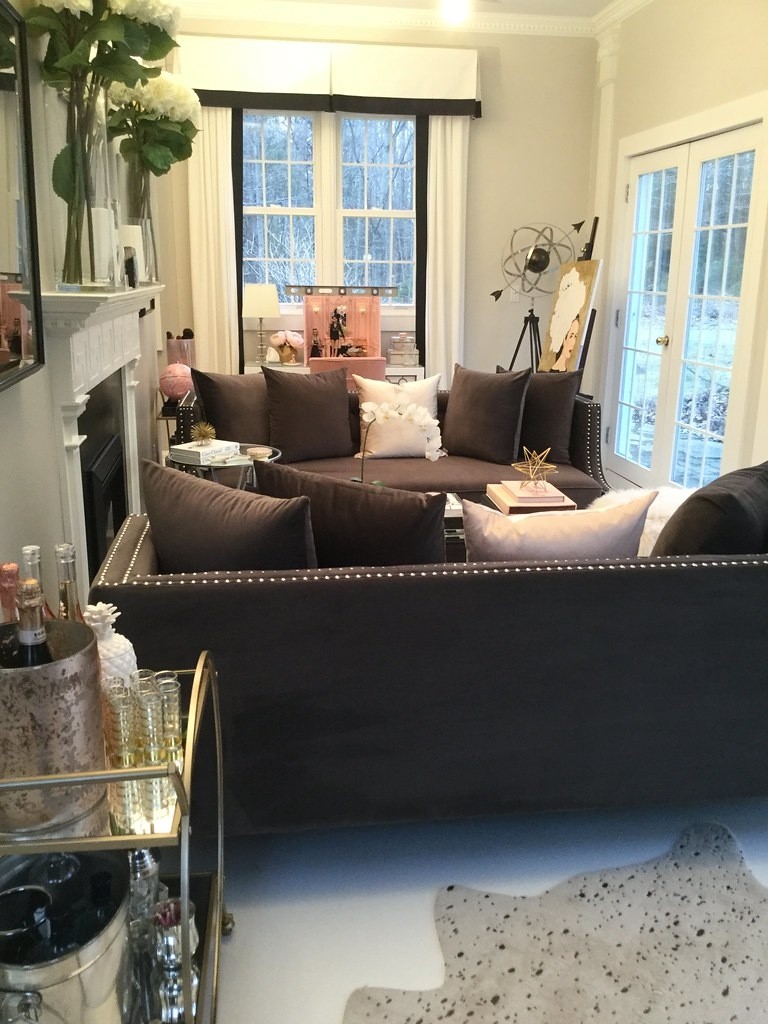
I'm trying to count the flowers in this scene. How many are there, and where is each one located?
[269,330,305,351]
[25,0,182,284]
[350,402,445,487]
[106,67,203,283]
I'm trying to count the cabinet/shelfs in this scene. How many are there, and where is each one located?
[1,650,236,1024]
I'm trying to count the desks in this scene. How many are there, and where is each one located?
[169,442,283,490]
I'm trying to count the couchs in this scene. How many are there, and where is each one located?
[172,385,616,511]
[92,513,768,841]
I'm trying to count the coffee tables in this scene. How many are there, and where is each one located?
[442,491,502,546]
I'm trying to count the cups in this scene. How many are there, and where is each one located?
[95,669,184,835]
[145,897,200,967]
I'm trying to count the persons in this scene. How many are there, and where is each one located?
[310,328,323,358]
[4,315,22,358]
[330,313,346,357]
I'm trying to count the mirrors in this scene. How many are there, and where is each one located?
[0,0,47,396]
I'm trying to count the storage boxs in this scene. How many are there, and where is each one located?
[391,337,415,352]
[387,347,419,366]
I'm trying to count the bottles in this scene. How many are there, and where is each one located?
[0,542,86,671]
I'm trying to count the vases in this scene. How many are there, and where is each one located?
[114,154,161,289]
[278,346,298,363]
[42,80,115,295]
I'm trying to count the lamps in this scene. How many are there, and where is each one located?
[241,282,283,365]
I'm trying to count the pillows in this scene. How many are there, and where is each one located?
[441,364,534,465]
[462,489,659,564]
[352,374,445,459]
[650,460,768,557]
[261,365,358,464]
[191,368,270,447]
[496,365,586,464]
[253,459,446,568]
[139,458,318,572]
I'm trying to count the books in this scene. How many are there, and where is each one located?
[170,437,241,466]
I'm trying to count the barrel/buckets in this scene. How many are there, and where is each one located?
[0,618,107,840]
[0,849,136,1024]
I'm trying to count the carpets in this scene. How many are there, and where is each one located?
[340,824,767,1021]
